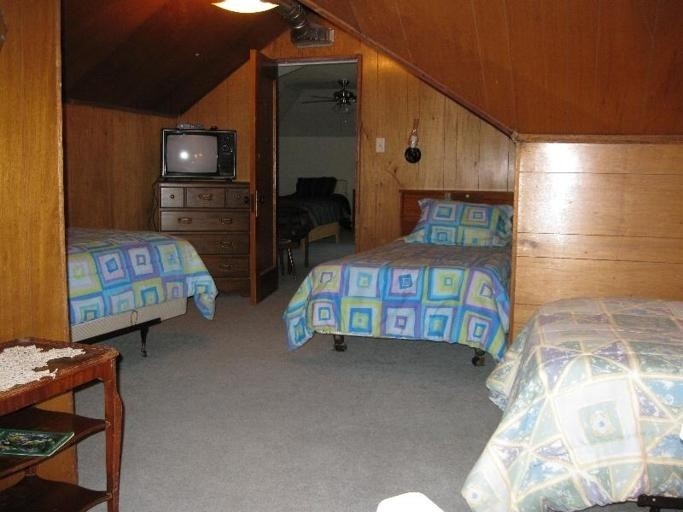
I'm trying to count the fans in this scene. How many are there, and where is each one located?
[302,79,357,104]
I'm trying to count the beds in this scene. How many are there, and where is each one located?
[281,188,514,367]
[459,296,683,512]
[277,179,352,250]
[66,223,219,358]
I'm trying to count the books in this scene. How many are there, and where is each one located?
[1,426,76,459]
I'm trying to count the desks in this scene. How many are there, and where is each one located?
[275,206,318,268]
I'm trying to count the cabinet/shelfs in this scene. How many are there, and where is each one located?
[0,336,124,512]
[150,181,253,297]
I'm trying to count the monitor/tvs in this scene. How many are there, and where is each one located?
[161,128,238,182]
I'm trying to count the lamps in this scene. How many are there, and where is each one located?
[331,97,353,114]
[211,0,334,49]
[404,117,421,163]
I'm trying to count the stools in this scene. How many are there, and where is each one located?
[276,239,295,276]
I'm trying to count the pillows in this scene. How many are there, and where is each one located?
[295,176,337,197]
[404,198,514,247]
